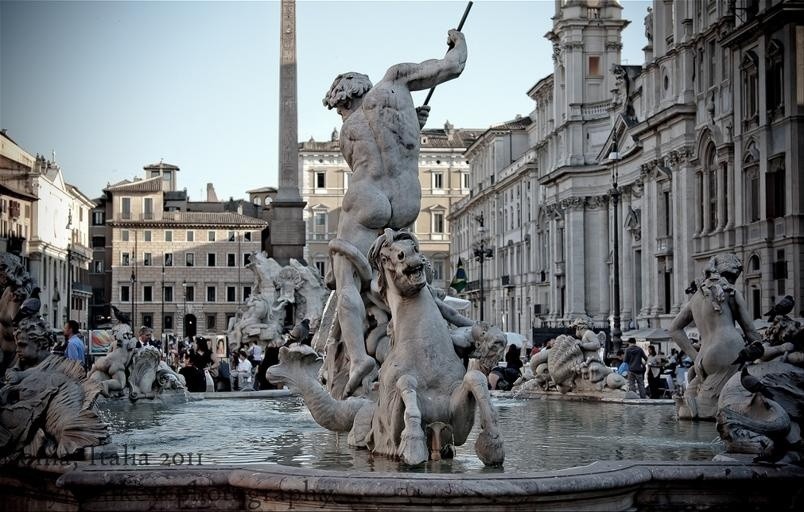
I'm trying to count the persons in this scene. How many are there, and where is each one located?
[61,321,678,400]
[3,315,105,457]
[671,253,761,378]
[322,28,468,399]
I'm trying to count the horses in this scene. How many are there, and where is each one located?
[358,224,506,470]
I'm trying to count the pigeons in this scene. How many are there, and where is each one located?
[738,365,775,409]
[731,340,766,368]
[283,318,310,348]
[763,293,796,325]
[752,430,794,467]
[110,304,133,326]
[12,285,44,326]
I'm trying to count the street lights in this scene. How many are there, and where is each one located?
[159,260,168,342]
[130,247,138,336]
[471,209,492,321]
[607,124,623,366]
[181,277,186,339]
[65,241,72,324]
